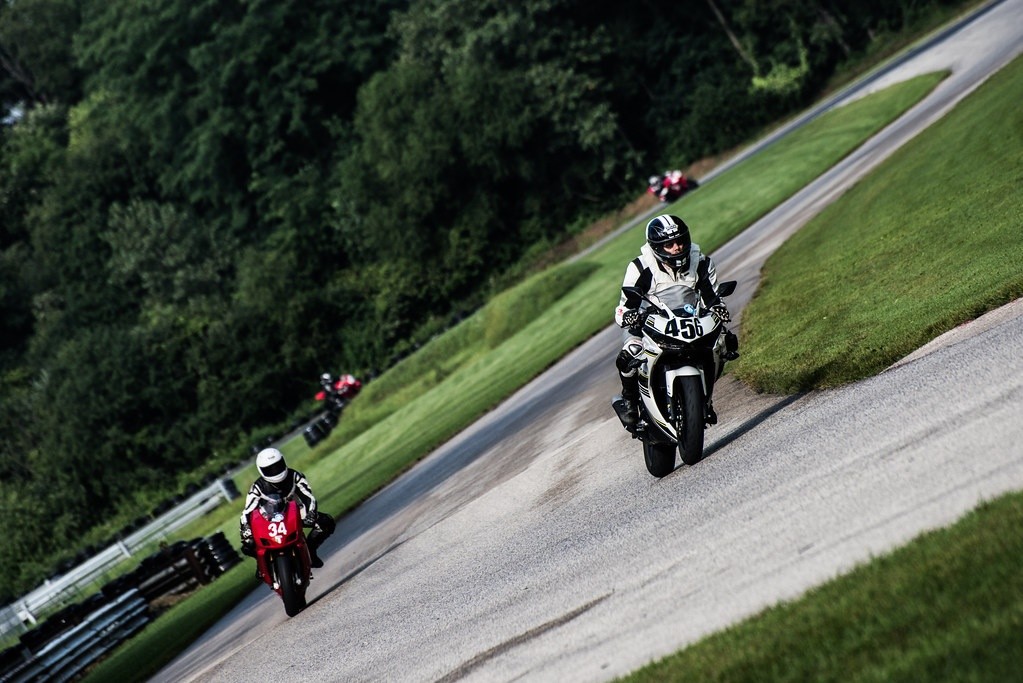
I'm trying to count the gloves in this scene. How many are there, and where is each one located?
[624,311,640,326]
[240,529,252,539]
[709,305,729,320]
[305,511,317,524]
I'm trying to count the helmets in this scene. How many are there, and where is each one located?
[645,215,691,271]
[256,448,288,483]
[323,374,332,383]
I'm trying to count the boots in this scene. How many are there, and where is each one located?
[619,372,638,415]
[706,405,717,424]
[307,528,329,568]
[255,569,263,579]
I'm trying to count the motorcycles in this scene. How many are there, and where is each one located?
[240,493,314,621]
[314,374,362,400]
[608,279,739,477]
[648,171,699,204]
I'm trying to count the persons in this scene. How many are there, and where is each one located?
[239,448,336,568]
[649,170,680,202]
[320,372,349,409]
[615,215,741,424]
